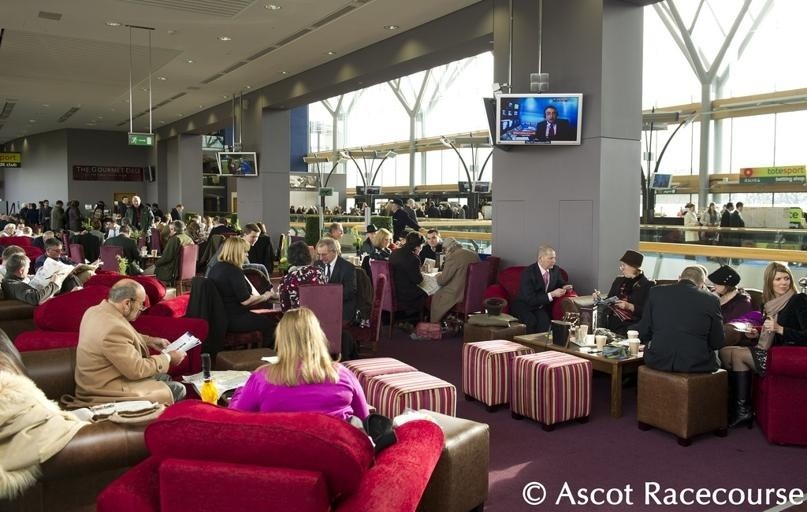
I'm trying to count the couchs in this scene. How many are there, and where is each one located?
[0,327,146,511]
[753,346,807,448]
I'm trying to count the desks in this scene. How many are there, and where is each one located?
[514,332,643,418]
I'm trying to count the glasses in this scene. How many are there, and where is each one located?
[47,247,64,253]
[126,301,148,313]
[618,264,629,271]
[315,250,334,257]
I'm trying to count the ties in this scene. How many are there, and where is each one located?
[542,270,549,290]
[326,264,331,278]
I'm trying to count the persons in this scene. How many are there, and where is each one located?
[236,157,252,174]
[388,231,426,315]
[315,222,343,254]
[228,306,369,430]
[708,265,753,321]
[104,227,145,276]
[511,245,573,334]
[697,265,710,292]
[0,195,239,238]
[0,245,26,281]
[290,202,367,217]
[362,224,380,252]
[419,230,446,263]
[315,237,358,358]
[279,241,330,314]
[381,198,490,232]
[34,238,97,292]
[2,253,62,306]
[370,228,393,260]
[208,236,280,348]
[143,221,195,283]
[429,236,480,323]
[592,249,657,335]
[205,224,269,280]
[536,106,572,140]
[248,223,274,274]
[719,260,807,429]
[74,279,187,405]
[638,266,725,373]
[677,202,745,247]
[35,232,80,273]
[228,159,236,174]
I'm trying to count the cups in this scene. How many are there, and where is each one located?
[596,335,608,349]
[629,339,640,356]
[574,327,581,342]
[151,249,157,257]
[627,330,640,340]
[352,257,360,266]
[579,325,588,344]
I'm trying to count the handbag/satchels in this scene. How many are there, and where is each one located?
[551,319,571,348]
[277,261,327,314]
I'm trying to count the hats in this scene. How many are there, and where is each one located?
[481,297,508,316]
[619,250,644,268]
[365,223,379,233]
[442,236,458,254]
[708,266,741,287]
[403,231,426,247]
[390,198,404,207]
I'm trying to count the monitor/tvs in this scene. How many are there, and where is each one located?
[145,164,155,183]
[217,151,258,177]
[494,91,585,148]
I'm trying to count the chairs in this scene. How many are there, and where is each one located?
[97,400,445,512]
[482,266,578,320]
[0,237,208,396]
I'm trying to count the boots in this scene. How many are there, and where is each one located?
[729,369,754,431]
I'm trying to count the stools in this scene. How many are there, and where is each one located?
[393,410,491,512]
[638,366,727,446]
[460,340,535,411]
[463,324,524,342]
[511,351,591,431]
[218,349,279,371]
[340,356,418,397]
[366,372,457,421]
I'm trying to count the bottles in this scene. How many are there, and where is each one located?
[200,354,218,405]
